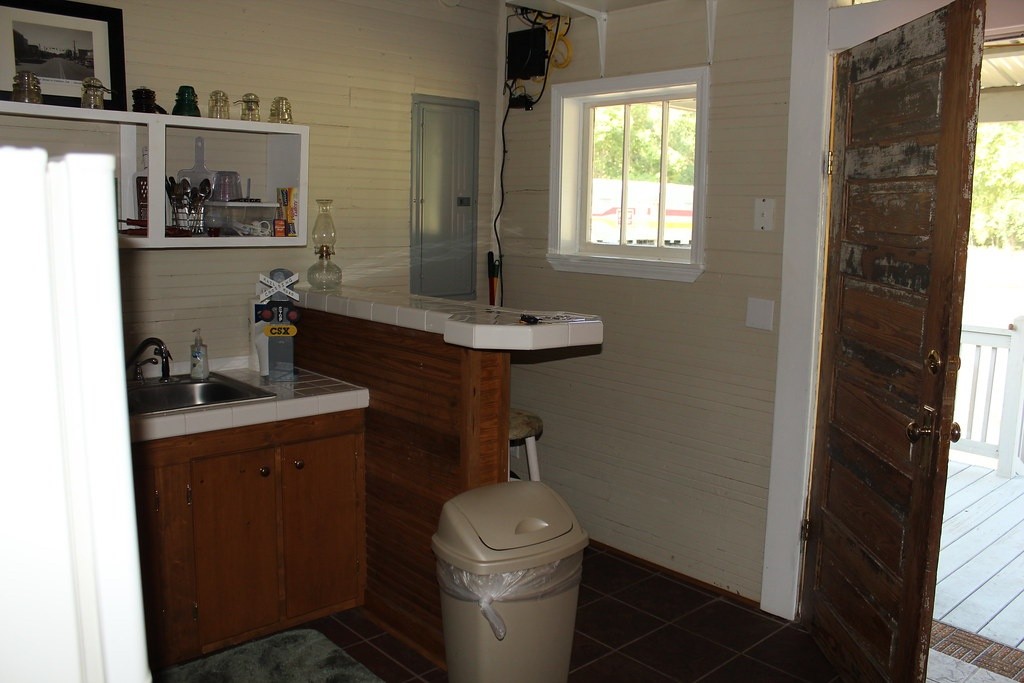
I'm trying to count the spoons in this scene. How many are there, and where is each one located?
[165,176,212,233]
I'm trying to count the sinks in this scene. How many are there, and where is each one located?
[126,371,278,419]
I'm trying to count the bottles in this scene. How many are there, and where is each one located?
[13,71,41,104]
[269,96,294,125]
[240,93,260,121]
[81,77,104,109]
[171,84,201,117]
[209,90,229,120]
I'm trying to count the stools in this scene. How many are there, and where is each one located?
[508,409,543,481]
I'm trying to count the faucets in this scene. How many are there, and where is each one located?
[125,337,172,386]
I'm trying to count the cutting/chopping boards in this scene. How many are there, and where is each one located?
[131,145,169,227]
[177,136,217,188]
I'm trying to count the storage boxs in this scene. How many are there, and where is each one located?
[203,201,280,231]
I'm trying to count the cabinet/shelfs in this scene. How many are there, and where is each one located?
[410,93,480,302]
[0,100,310,249]
[131,409,368,672]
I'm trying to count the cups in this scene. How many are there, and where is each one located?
[213,171,243,201]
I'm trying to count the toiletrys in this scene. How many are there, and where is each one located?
[190,328,209,379]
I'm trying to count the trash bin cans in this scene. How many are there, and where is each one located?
[431,479,590,683]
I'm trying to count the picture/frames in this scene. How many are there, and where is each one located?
[0,0,127,111]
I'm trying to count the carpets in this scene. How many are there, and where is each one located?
[155,629,383,683]
